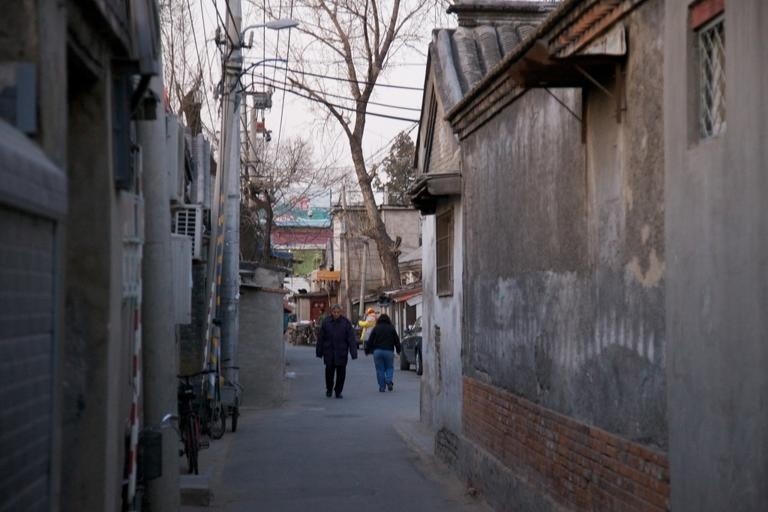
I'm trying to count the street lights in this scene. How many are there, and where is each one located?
[219,15,298,415]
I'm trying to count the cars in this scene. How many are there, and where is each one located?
[397,314,423,375]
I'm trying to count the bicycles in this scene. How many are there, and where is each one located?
[177,370,215,474]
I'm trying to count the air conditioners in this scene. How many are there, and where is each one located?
[174,204,204,261]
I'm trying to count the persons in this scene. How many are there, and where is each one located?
[365,314,401,392]
[316,304,357,398]
[313,308,325,344]
[359,308,375,351]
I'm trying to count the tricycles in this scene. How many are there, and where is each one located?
[207,358,241,440]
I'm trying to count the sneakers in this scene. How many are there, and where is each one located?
[379,383,393,392]
[326,388,343,398]
